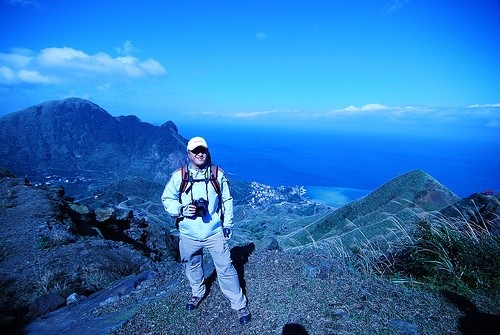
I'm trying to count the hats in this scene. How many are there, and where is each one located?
[187,137,208,151]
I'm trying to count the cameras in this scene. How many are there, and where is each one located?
[192,199,209,217]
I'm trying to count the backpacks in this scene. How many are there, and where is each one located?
[175,165,224,230]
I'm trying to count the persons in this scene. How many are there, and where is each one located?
[161,136,251,324]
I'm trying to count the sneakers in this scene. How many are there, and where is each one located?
[236,305,251,325]
[186,290,208,310]
